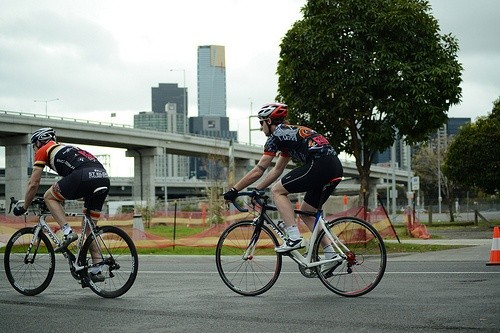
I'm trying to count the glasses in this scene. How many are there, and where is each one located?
[260,119,268,125]
[33,144,35,148]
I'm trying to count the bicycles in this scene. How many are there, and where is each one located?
[4,197,139,299]
[215,189,387,297]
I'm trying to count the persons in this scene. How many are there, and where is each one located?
[13,127,110,275]
[224,102,344,273]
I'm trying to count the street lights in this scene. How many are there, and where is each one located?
[169,68,188,134]
[33,98,60,118]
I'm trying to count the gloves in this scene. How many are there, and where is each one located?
[13,206,27,216]
[224,187,239,203]
[251,188,265,200]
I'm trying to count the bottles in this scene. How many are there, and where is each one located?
[53,226,64,242]
[277,220,288,234]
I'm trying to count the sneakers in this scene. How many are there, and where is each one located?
[54,231,78,252]
[321,254,341,276]
[79,272,105,284]
[274,237,306,252]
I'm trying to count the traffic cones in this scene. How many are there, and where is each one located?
[486,227,500,265]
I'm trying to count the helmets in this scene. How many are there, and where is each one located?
[31,128,56,143]
[258,103,288,119]
